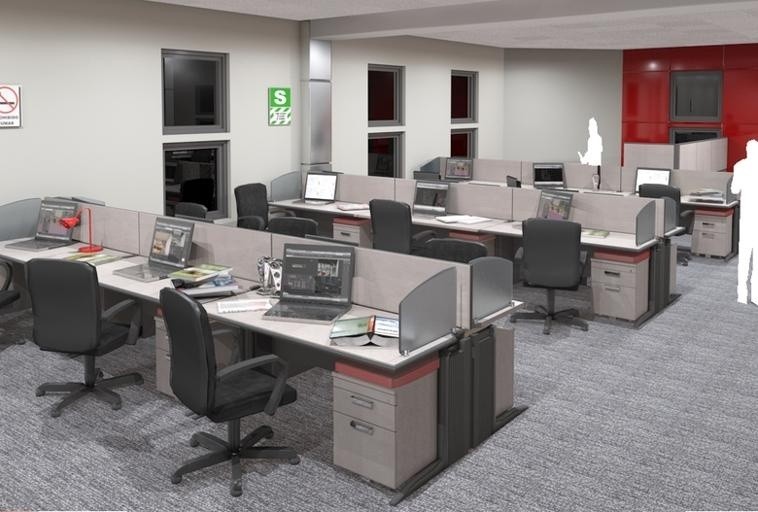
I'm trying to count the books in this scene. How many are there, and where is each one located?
[166,261,231,279]
[688,197,724,204]
[688,189,722,198]
[60,252,120,268]
[330,315,399,348]
[584,230,609,239]
[337,203,369,211]
[436,213,470,222]
[458,216,493,225]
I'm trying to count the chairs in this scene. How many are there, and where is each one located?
[0,182,528,506]
[269,170,740,336]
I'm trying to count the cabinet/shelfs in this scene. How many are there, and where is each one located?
[718,67,757,172]
[618,72,670,168]
[622,40,757,73]
